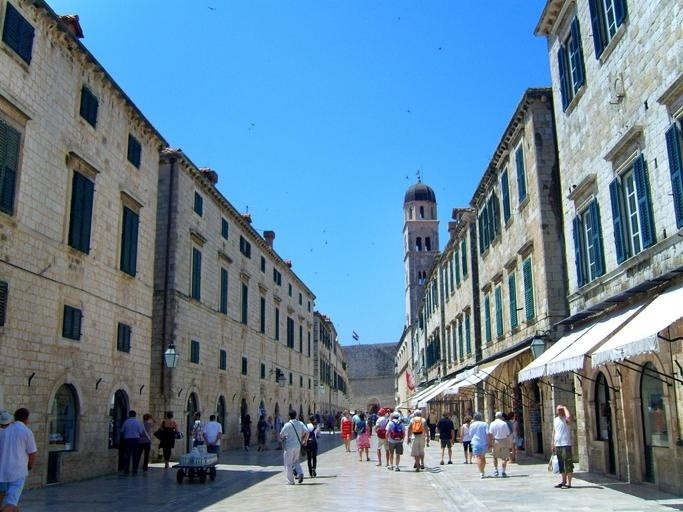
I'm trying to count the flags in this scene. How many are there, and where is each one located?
[351,331,359,341]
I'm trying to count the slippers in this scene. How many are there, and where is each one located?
[561,484,571,489]
[555,482,565,488]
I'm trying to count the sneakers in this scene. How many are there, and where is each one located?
[298,474,303,483]
[479,469,507,478]
[439,460,444,465]
[284,482,295,485]
[464,461,468,464]
[448,460,453,464]
[312,469,317,477]
[376,462,427,472]
[366,457,370,461]
[359,459,362,462]
[345,449,351,451]
[469,462,472,464]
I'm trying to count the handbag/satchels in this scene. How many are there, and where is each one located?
[154,428,161,439]
[301,445,306,455]
[551,455,560,473]
[547,452,554,472]
[377,429,387,437]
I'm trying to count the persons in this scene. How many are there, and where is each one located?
[240,407,518,478]
[135,413,156,473]
[201,414,222,463]
[550,404,575,489]
[117,409,143,479]
[278,409,310,486]
[157,410,184,469]
[0,407,39,512]
[190,411,204,448]
[0,411,13,430]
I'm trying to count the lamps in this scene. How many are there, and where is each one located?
[529,329,551,359]
[150,336,179,367]
[268,364,288,388]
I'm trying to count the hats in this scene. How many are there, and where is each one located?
[0,412,15,425]
[379,407,386,415]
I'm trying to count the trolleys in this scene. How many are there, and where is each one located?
[170,463,217,485]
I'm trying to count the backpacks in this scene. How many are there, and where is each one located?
[390,420,404,440]
[303,428,317,451]
[411,416,424,436]
